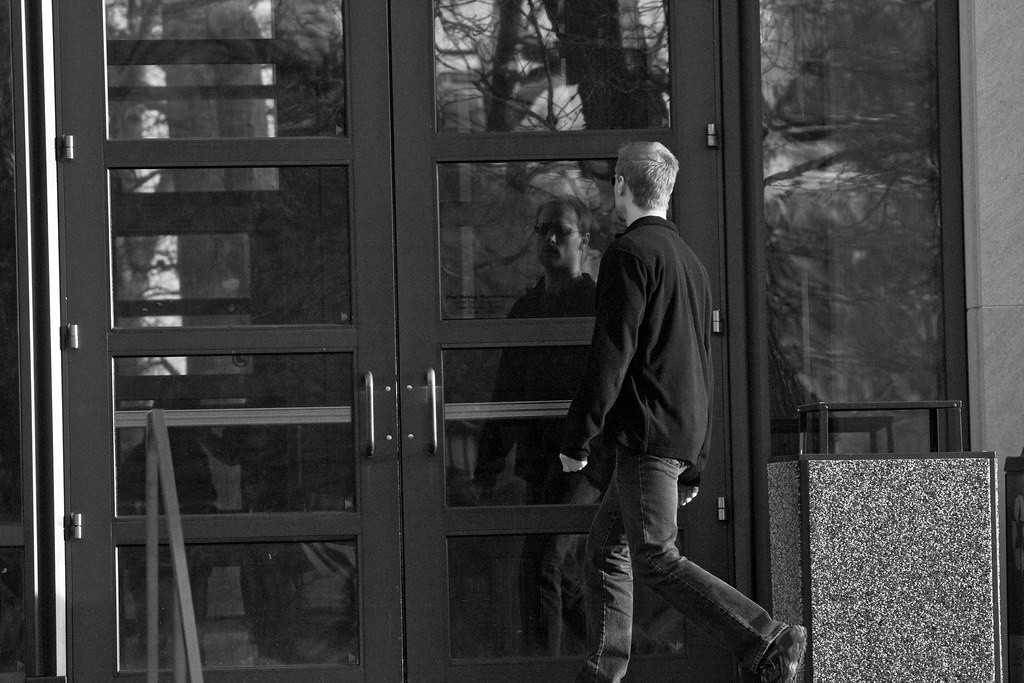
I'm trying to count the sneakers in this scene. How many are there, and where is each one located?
[757,624,808,683]
[649,638,684,656]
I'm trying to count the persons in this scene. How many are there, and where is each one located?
[558,140,806,683]
[472,196,658,658]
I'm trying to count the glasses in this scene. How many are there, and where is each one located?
[534,224,580,239]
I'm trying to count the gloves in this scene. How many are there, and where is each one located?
[560,453,589,473]
[677,483,699,510]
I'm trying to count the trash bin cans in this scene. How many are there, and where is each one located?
[763,399,1007,682]
